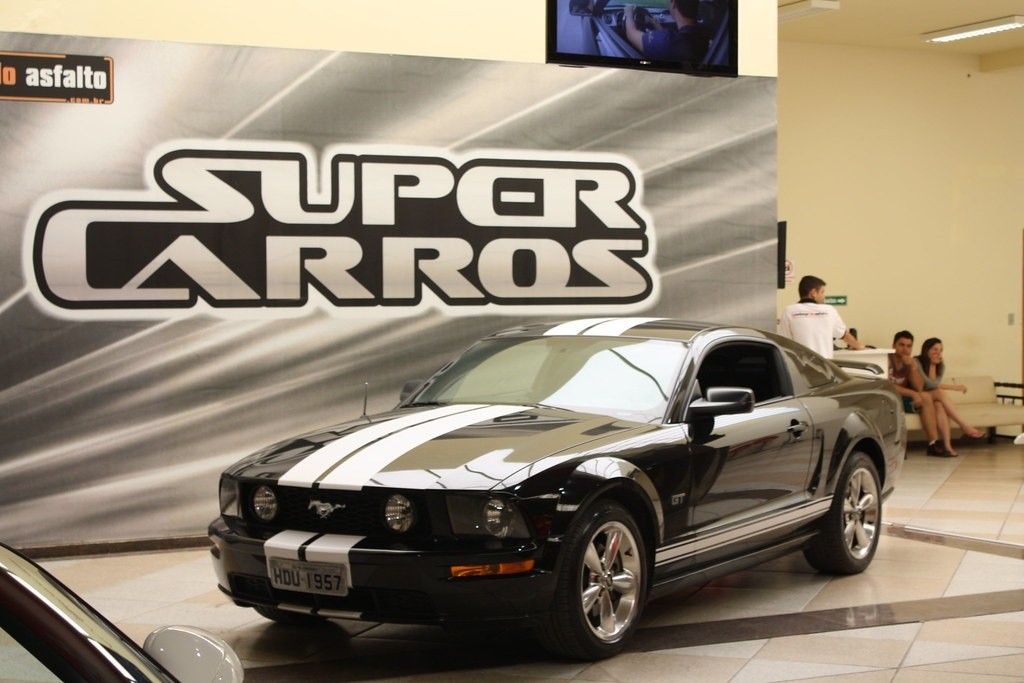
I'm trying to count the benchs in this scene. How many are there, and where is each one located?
[896,375,1024,461]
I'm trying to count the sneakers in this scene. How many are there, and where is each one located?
[927,440,951,457]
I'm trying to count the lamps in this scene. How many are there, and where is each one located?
[921,15,1024,42]
[778,0,840,23]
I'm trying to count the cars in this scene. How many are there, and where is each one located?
[569,0,730,66]
[206,318,909,662]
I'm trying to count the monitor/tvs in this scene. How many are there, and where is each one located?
[545,0,740,77]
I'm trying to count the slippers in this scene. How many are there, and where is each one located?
[962,430,985,439]
[951,451,958,457]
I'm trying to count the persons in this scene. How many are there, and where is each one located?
[888,331,985,458]
[779,276,865,359]
[624,0,709,63]
